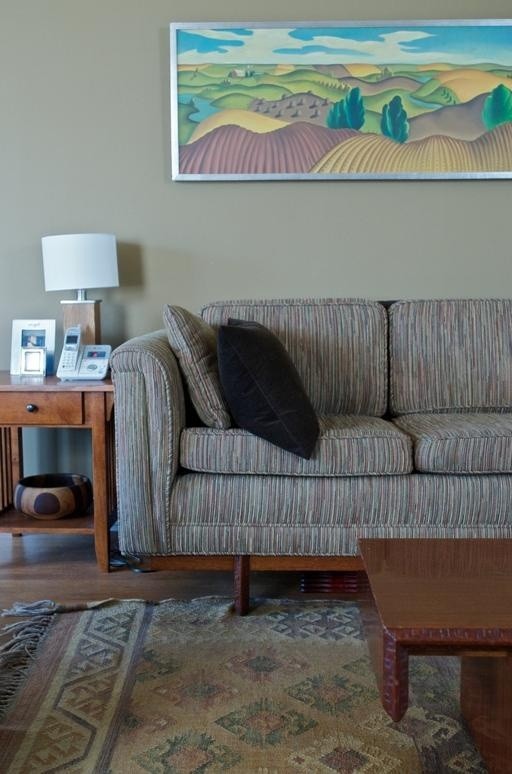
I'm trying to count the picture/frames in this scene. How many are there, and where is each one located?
[19,346,47,377]
[169,18,511,182]
[10,319,57,376]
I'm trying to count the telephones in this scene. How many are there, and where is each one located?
[55,325,112,380]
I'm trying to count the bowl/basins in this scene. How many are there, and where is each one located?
[12,471,93,520]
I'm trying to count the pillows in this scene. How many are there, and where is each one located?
[389,298,511,415]
[162,303,231,429]
[199,298,388,416]
[217,317,320,460]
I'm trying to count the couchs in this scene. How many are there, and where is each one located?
[108,297,511,617]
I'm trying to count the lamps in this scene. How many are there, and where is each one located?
[41,232,119,345]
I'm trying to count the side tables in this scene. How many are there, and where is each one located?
[0,369,117,574]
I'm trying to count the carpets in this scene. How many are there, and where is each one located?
[1,595,491,774]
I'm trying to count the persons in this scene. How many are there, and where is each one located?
[23,334,40,346]
